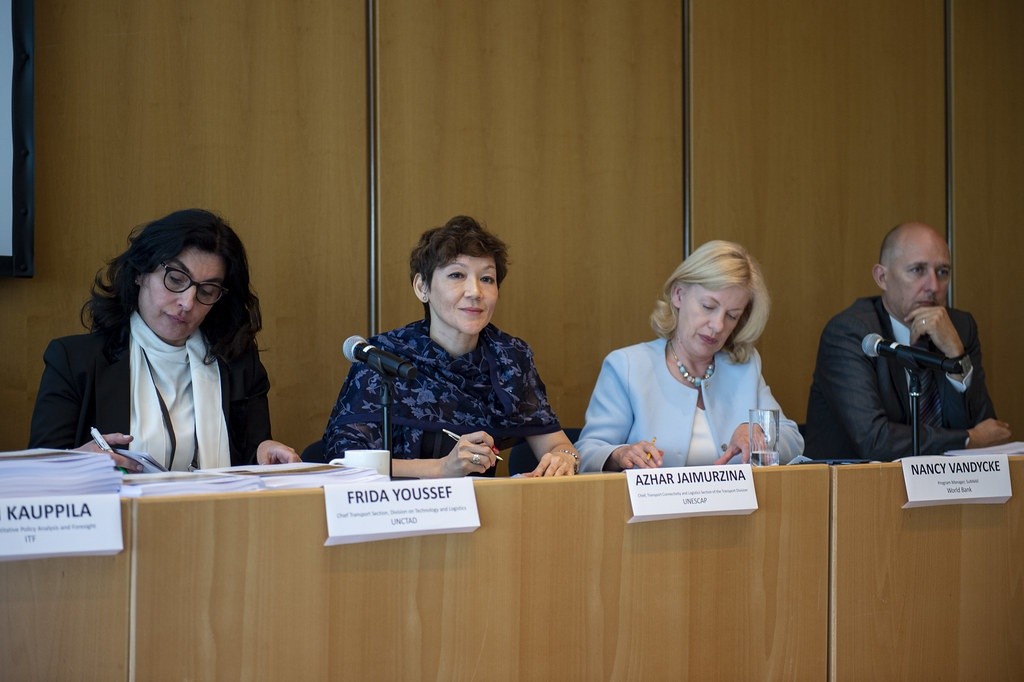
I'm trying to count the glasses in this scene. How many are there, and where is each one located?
[159,262,229,305]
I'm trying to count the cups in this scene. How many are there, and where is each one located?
[748,408,780,467]
[329,449,391,475]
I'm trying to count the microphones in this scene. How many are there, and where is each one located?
[862,333,963,374]
[343,335,417,380]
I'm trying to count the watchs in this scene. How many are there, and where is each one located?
[560,449,580,473]
[946,353,972,376]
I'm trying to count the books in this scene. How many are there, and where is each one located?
[1,447,390,499]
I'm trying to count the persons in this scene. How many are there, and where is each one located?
[26,207,303,474]
[574,240,806,474]
[804,221,1013,462]
[299,215,580,478]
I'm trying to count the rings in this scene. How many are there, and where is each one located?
[472,454,480,464]
[922,317,926,325]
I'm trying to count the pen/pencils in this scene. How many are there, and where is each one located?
[89,426,129,475]
[441,428,506,462]
[645,436,657,463]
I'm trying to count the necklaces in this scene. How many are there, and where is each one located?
[668,341,715,387]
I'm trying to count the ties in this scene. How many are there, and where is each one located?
[911,335,942,430]
